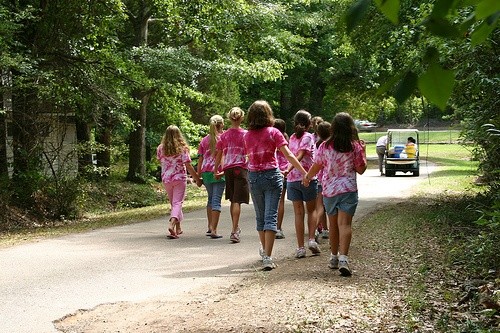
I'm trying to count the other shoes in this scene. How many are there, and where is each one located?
[259,247,264,257]
[295,249,306,258]
[309,241,322,254]
[261,260,276,270]
[338,260,352,275]
[318,229,323,233]
[322,230,329,238]
[229,227,242,243]
[381,173,385,176]
[275,230,285,238]
[327,259,339,269]
[315,230,319,241]
[206,230,223,238]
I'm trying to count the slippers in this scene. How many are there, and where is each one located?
[166,234,179,239]
[177,229,183,235]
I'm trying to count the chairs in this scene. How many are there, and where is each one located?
[394,146,404,157]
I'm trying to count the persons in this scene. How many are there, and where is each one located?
[405,137,415,146]
[157,125,202,239]
[197,115,228,240]
[376,135,391,176]
[243,101,308,269]
[213,106,249,242]
[273,110,331,258]
[304,111,368,276]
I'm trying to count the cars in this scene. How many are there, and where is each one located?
[355,119,379,132]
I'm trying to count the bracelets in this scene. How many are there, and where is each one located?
[305,174,310,181]
